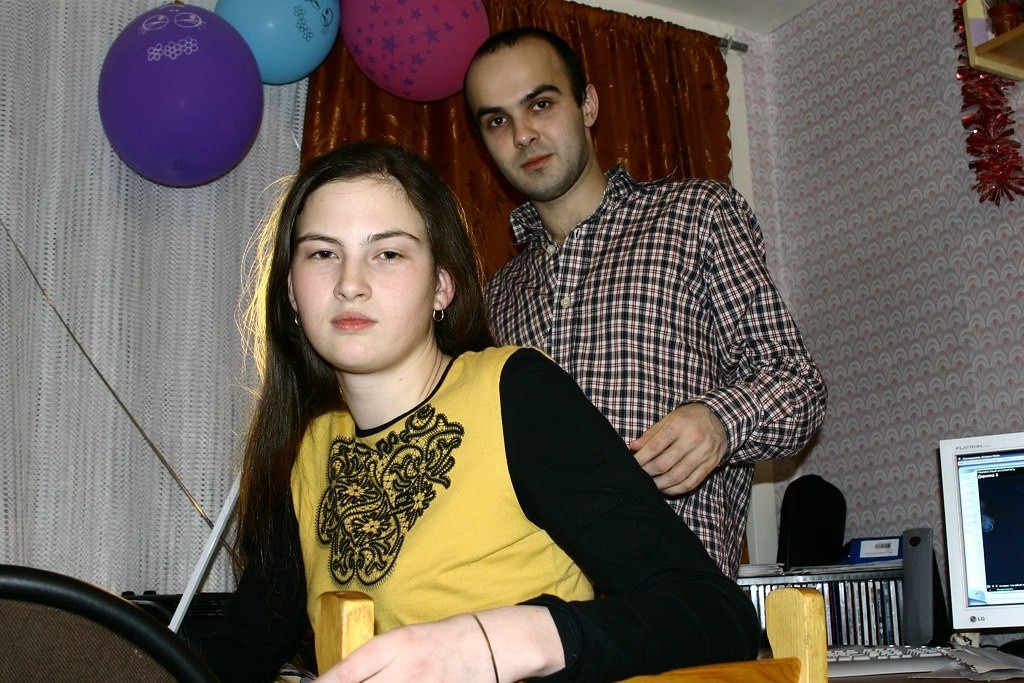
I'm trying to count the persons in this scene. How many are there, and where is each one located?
[482,25,826,583]
[205,138,761,683]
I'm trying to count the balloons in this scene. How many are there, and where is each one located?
[341,1,494,103]
[214,0,341,85]
[96,0,263,188]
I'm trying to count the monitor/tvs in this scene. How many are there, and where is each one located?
[937,432,1023,658]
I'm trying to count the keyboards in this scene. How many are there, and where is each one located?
[826,644,965,680]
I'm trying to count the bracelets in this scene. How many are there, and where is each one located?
[466,610,502,683]
